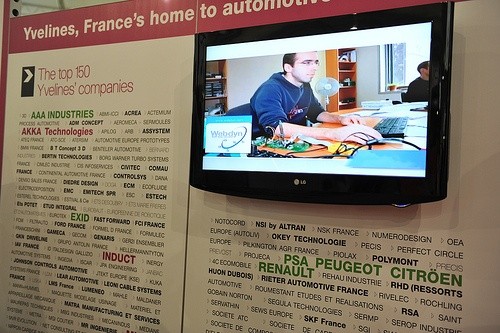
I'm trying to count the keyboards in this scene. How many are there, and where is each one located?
[374,116,410,138]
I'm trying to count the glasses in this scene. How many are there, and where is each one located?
[296,62,320,67]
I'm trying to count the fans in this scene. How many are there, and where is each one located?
[315,77,340,112]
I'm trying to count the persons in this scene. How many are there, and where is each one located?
[407,61,429,101]
[248,53,384,144]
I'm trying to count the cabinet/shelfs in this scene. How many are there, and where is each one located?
[206,60,229,116]
[325,48,358,114]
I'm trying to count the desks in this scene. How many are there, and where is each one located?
[256,101,429,161]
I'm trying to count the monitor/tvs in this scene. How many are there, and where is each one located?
[188,1,456,208]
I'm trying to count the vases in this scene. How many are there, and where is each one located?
[389,86,396,91]
